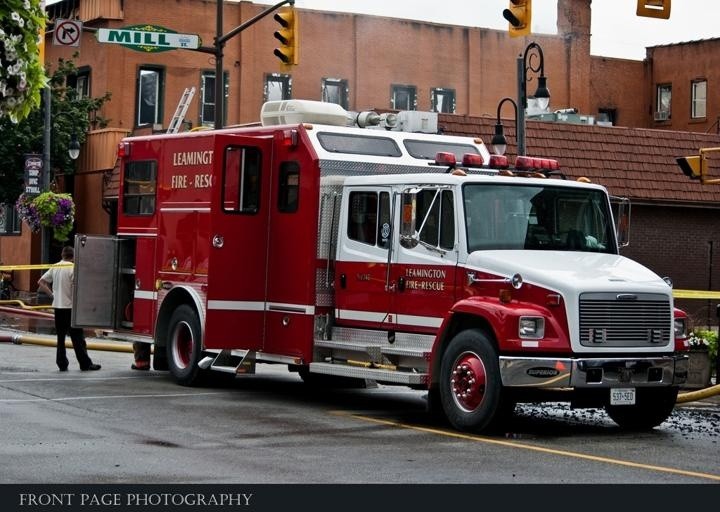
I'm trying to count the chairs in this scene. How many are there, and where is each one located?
[354,213,377,245]
[525,223,549,243]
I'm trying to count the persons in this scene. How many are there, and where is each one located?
[131,341,152,370]
[38,244,101,372]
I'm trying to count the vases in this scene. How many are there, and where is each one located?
[680,350,713,387]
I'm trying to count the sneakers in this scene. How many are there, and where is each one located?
[82,364,101,370]
[131,363,150,370]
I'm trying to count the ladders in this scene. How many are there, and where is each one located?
[165,86,196,134]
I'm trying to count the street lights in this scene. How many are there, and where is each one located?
[492,41,551,162]
[37,86,80,301]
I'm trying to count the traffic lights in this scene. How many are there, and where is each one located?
[272,6,299,71]
[675,154,709,180]
[503,0,533,38]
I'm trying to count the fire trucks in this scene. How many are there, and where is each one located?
[67,100,690,436]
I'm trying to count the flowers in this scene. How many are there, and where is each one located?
[14,192,76,242]
[689,326,719,359]
[0,0,53,123]
